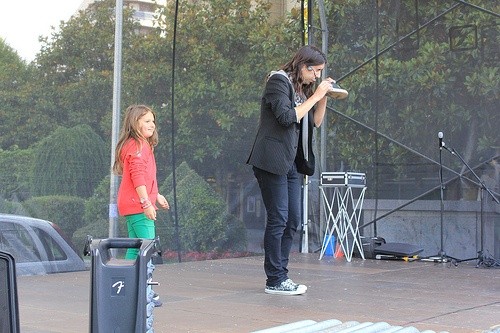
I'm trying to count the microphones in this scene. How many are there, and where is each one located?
[437,131,444,150]
[308,66,316,76]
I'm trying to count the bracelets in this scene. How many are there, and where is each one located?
[140,203,153,209]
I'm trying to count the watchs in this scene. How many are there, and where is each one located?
[138,198,148,205]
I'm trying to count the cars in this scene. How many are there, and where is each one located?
[0,212,89,276]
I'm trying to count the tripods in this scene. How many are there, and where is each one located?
[415,149,460,263]
[457,186,500,268]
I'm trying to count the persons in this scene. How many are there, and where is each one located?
[111,104,170,307]
[244,44,337,297]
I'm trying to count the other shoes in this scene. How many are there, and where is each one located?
[153,294,160,300]
[324,79,348,100]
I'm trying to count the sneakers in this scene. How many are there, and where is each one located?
[265,278,307,295]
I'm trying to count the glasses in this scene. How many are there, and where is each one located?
[310,66,320,84]
